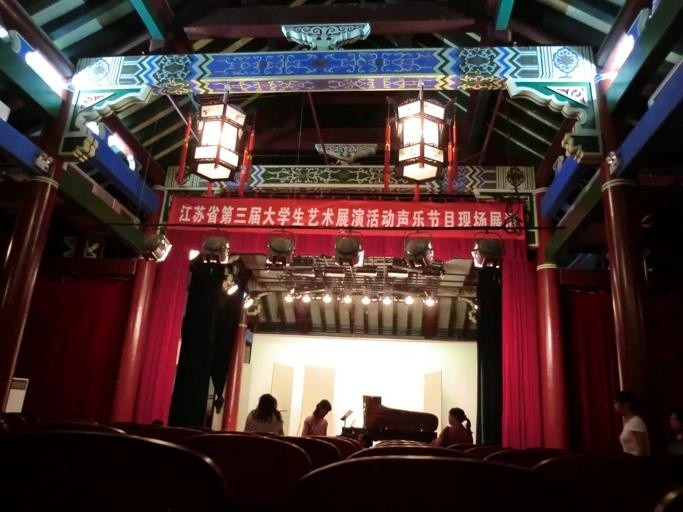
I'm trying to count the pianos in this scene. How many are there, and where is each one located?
[363,395,438,448]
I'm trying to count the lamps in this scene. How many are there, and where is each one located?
[385,90,457,184]
[141,224,504,270]
[221,266,254,310]
[189,93,259,182]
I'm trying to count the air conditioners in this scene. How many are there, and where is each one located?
[5,377,29,413]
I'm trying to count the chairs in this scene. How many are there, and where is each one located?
[294,439,599,510]
[1,425,361,510]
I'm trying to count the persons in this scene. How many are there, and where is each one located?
[300,400,331,436]
[613,389,651,457]
[659,411,683,454]
[244,393,283,436]
[430,407,474,447]
